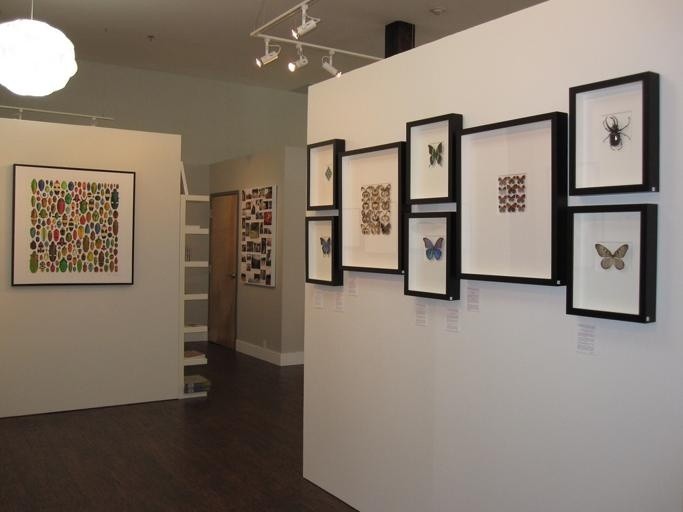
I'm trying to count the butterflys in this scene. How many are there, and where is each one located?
[595,244,628,270]
[361,184,391,235]
[320,237,330,257]
[498,175,525,213]
[423,238,444,260]
[428,143,442,168]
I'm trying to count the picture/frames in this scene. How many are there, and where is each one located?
[304,214,343,286]
[337,140,410,274]
[405,114,463,205]
[11,164,136,287]
[564,203,657,323]
[402,211,455,301]
[306,138,345,211]
[454,110,567,287]
[568,71,660,196]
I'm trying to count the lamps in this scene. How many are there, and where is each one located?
[0,0,78,98]
[255,38,342,78]
[292,19,317,41]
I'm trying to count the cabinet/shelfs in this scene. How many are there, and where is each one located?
[181,195,210,399]
